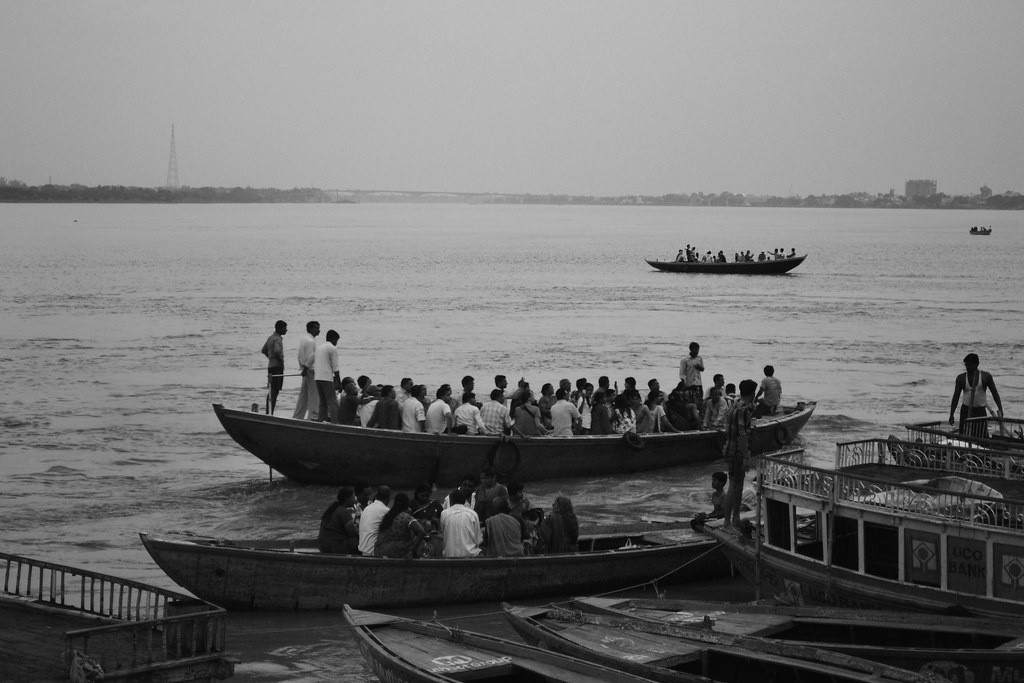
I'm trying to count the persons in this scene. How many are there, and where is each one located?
[300,329,344,426]
[679,341,704,411]
[262,318,288,414]
[675,244,796,262]
[316,464,580,560]
[292,321,321,421]
[693,471,728,520]
[721,379,759,535]
[339,361,782,437]
[948,353,1004,447]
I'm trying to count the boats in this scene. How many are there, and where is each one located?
[211,400,818,489]
[644,253,808,275]
[970,229,992,235]
[137,499,1024,683]
